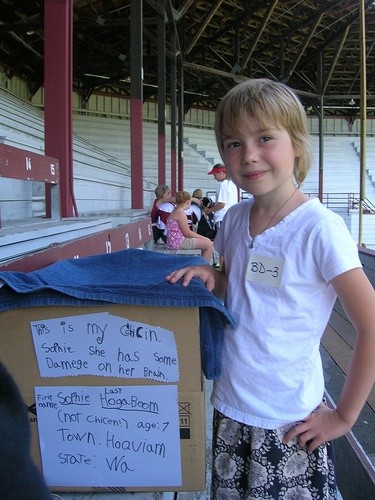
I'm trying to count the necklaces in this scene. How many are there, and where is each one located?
[246,188,297,248]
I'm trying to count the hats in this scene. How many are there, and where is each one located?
[207,168,227,175]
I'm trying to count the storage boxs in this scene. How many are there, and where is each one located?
[0,306,206,493]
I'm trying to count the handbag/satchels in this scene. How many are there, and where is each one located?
[197,205,218,241]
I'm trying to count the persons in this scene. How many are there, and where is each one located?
[150,164,238,268]
[166,77,375,500]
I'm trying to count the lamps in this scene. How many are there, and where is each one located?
[348,98,355,105]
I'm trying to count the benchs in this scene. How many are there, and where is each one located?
[0,85,375,500]
